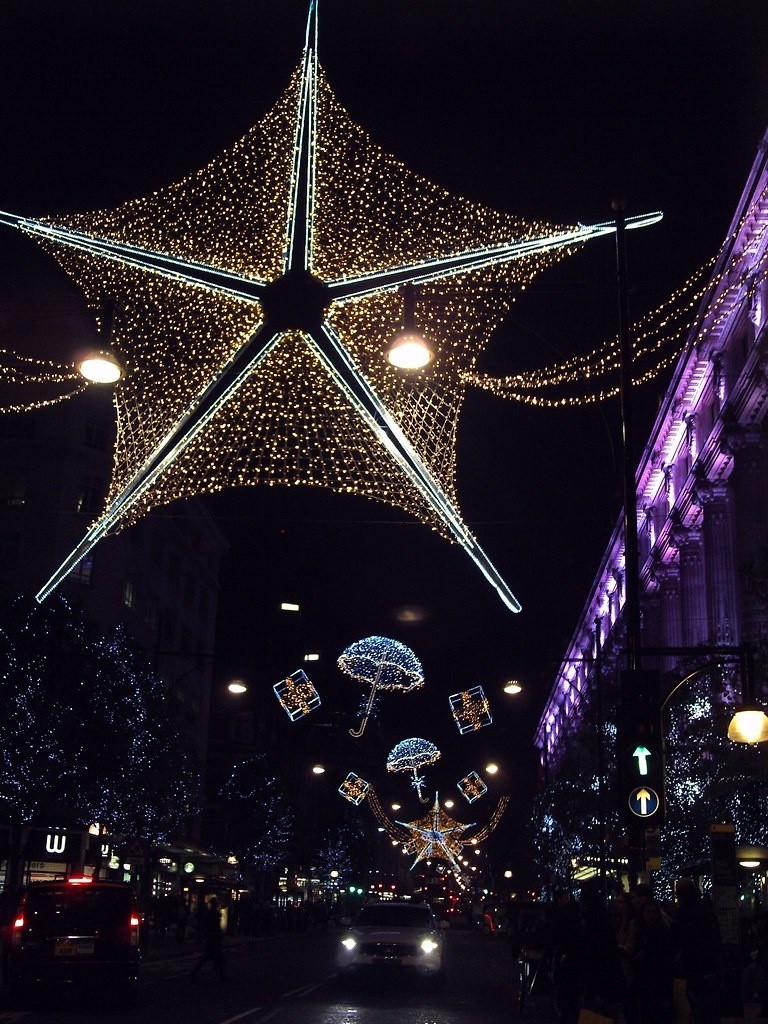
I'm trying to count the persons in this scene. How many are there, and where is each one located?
[1,878,345,961]
[529,882,768,1023]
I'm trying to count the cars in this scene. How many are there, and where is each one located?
[9,879,144,993]
[337,900,450,990]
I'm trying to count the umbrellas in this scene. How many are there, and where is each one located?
[386,737,440,803]
[337,634,425,737]
